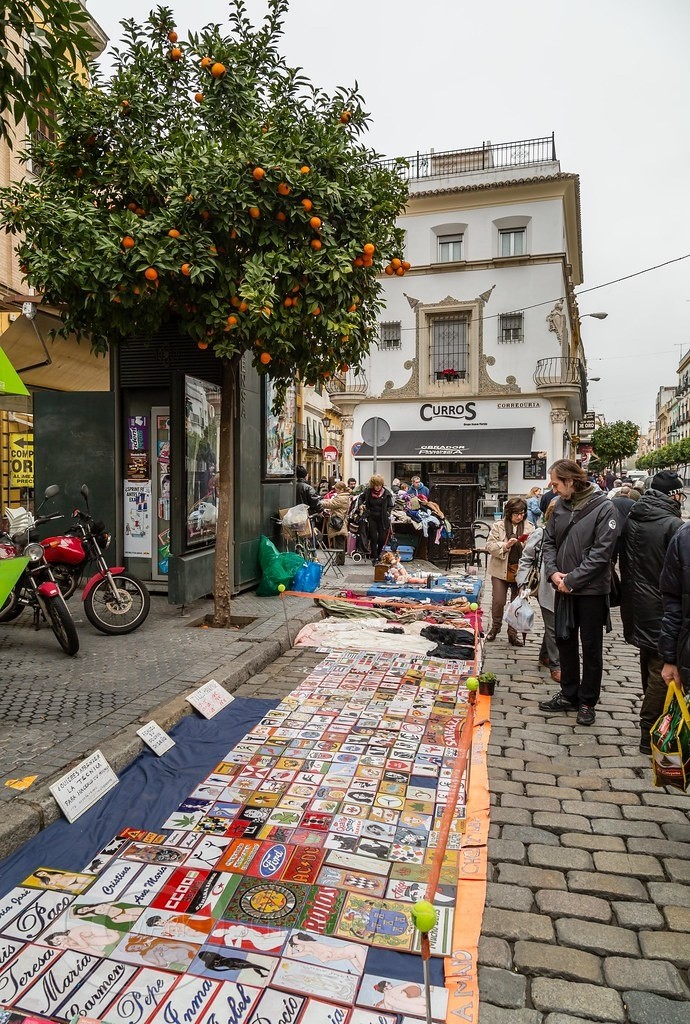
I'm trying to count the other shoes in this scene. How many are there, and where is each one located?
[551,670,561,682]
[486,627,501,640]
[332,561,344,565]
[639,739,652,754]
[539,656,550,667]
[509,635,523,645]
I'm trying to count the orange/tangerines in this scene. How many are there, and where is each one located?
[10,31,411,386]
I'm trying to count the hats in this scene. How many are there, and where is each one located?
[613,479,622,484]
[630,490,641,500]
[295,465,307,479]
[651,471,684,496]
[621,487,630,494]
[633,481,645,492]
[321,476,327,481]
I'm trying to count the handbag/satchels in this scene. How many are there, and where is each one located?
[329,517,343,531]
[503,564,540,633]
[649,679,690,793]
[349,523,359,532]
[291,561,324,593]
[610,570,621,607]
[390,525,398,551]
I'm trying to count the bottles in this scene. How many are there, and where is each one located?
[431,574,435,588]
[427,574,432,589]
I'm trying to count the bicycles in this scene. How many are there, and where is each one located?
[269,512,319,562]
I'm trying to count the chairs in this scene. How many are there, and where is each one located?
[467,521,491,567]
[446,526,474,571]
[314,527,345,578]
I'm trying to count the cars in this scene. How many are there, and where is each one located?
[615,469,628,479]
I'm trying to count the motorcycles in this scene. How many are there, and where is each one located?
[0,484,151,656]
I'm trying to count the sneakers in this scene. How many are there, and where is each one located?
[540,691,579,711]
[576,704,596,725]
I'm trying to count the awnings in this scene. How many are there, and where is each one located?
[352,427,536,462]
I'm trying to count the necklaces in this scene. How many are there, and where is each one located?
[373,489,382,496]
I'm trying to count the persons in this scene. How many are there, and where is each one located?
[486,497,535,647]
[621,469,690,755]
[295,465,324,561]
[515,495,562,683]
[319,481,350,566]
[391,478,408,493]
[522,468,647,575]
[406,475,430,500]
[538,458,619,726]
[358,474,394,567]
[345,478,356,494]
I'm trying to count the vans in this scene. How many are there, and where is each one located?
[626,470,649,483]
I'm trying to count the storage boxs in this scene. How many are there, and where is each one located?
[397,546,414,562]
[279,506,312,540]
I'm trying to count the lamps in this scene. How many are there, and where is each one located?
[585,376,601,381]
[576,313,610,324]
[322,417,343,435]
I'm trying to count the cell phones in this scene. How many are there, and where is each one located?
[517,534,529,542]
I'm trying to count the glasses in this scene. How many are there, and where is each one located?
[679,493,688,502]
[513,512,525,517]
